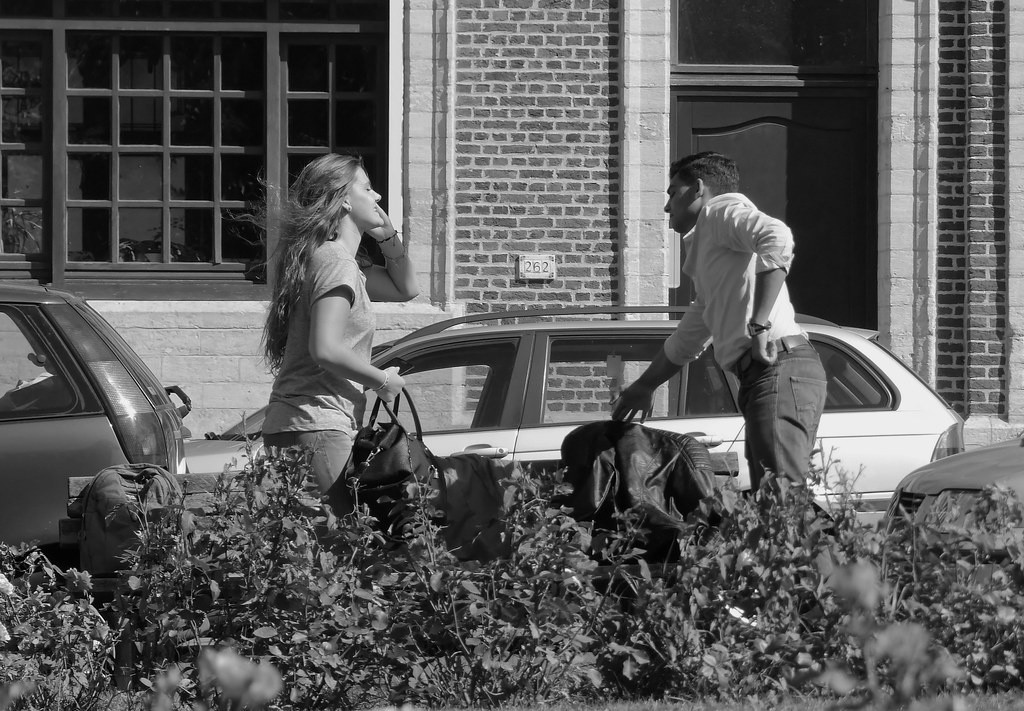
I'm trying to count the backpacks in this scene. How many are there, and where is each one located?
[73,463,194,578]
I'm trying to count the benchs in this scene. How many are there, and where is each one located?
[59,452,739,692]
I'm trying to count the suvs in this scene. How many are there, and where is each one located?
[179,306,965,546]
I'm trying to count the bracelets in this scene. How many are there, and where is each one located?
[376,230,397,246]
[383,245,405,261]
[373,371,389,391]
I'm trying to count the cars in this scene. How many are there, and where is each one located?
[871,431,1024,593]
[1,282,190,604]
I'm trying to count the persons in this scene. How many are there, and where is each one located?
[264,153,420,515]
[609,150,831,581]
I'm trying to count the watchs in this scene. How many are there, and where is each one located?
[747,321,771,335]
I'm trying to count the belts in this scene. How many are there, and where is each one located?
[734,335,810,382]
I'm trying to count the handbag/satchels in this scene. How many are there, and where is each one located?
[342,387,447,536]
